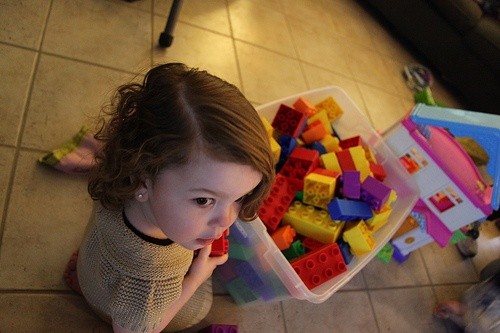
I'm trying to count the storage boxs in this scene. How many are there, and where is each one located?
[212,84,421,310]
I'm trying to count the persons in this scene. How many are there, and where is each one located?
[64,62,277,332]
[428,256,500,333]
